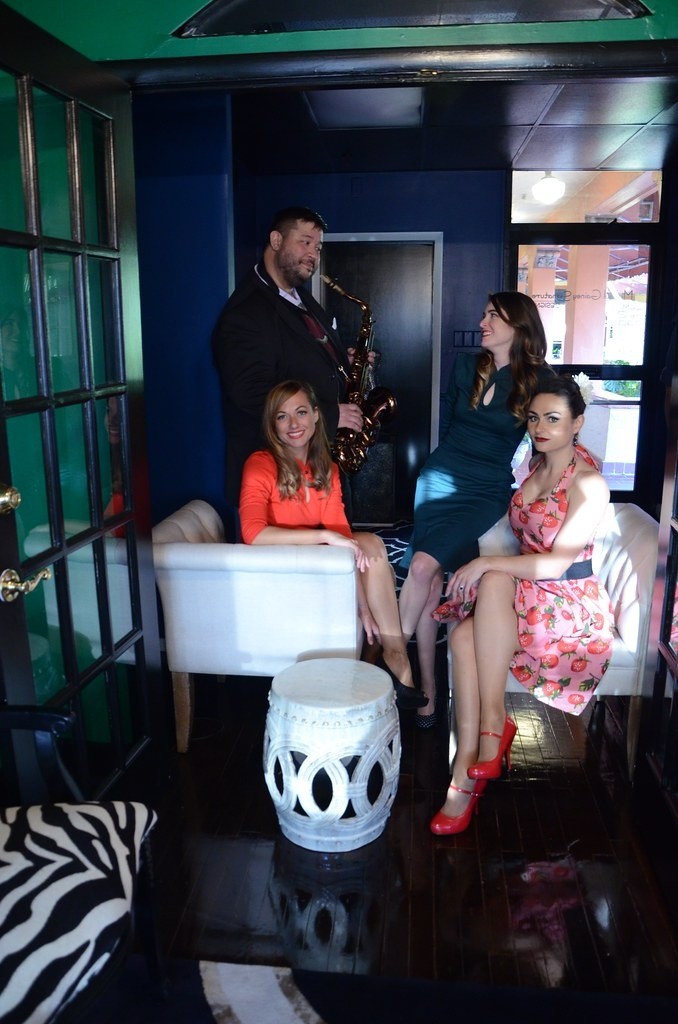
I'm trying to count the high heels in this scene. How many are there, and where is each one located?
[467,716,518,781]
[431,779,487,834]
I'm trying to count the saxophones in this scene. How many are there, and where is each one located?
[320,276,398,476]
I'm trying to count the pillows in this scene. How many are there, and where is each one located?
[0,798,157,1023]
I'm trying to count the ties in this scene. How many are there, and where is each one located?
[298,305,340,369]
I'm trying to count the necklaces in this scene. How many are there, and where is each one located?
[285,287,294,295]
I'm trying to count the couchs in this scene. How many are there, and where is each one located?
[446,500,663,762]
[155,501,407,756]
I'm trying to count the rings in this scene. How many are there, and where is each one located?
[458,586,464,592]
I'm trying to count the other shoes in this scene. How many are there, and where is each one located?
[373,653,429,709]
[414,692,439,729]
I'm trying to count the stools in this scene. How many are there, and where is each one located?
[263,659,404,855]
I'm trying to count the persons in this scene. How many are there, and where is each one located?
[430,370,614,835]
[238,380,432,711]
[210,206,377,530]
[96,397,128,538]
[396,290,555,729]
